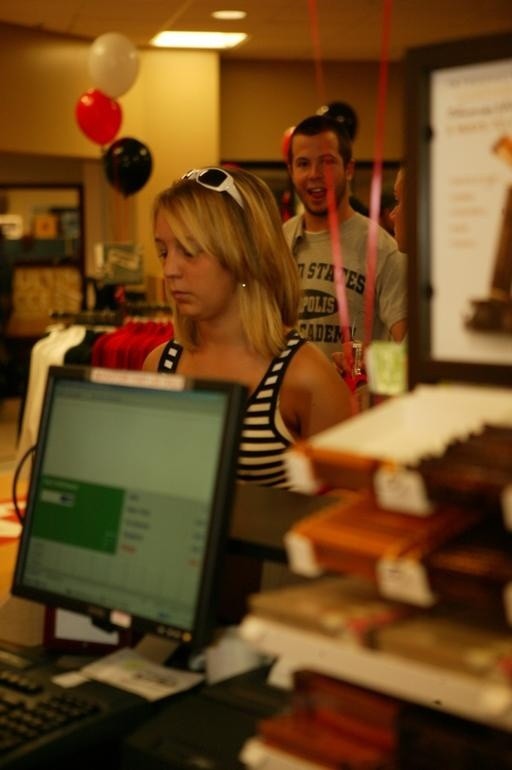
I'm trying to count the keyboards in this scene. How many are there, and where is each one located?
[0,646,142,760]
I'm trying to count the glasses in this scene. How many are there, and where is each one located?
[178,167,244,210]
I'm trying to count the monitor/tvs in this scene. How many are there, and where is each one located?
[9,366,248,644]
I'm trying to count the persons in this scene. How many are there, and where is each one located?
[137,161,358,500]
[388,165,411,254]
[275,113,414,407]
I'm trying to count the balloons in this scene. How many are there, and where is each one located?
[102,136,152,197]
[70,89,124,145]
[86,30,143,98]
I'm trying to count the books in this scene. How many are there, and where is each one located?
[228,386,512,770]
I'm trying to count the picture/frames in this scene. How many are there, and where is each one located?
[402,28,511,388]
